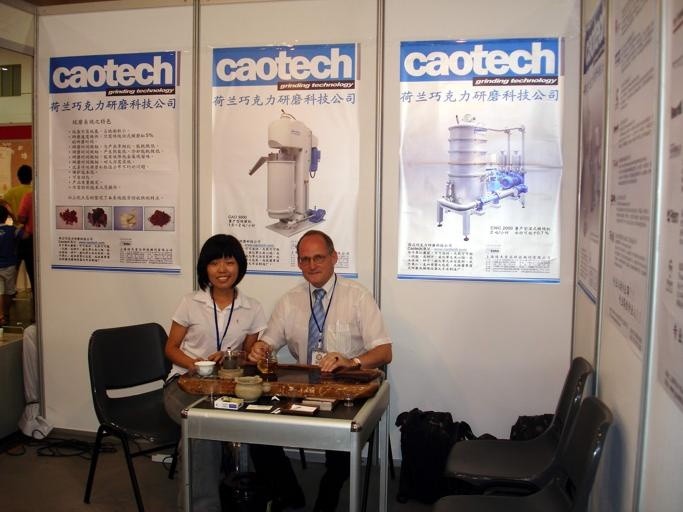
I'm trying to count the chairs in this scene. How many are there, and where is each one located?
[433,396,613,512]
[446,357,593,496]
[84,323,182,512]
[299,366,395,512]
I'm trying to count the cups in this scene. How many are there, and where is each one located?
[255,344,280,377]
[263,382,271,393]
[193,360,217,378]
[233,374,264,404]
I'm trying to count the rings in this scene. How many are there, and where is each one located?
[335,357,338,361]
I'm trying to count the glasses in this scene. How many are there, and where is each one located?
[298,253,325,265]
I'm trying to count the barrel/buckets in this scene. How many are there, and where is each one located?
[449,125,487,206]
[266,160,295,219]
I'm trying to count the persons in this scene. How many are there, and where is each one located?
[163,233,268,511]
[246,231,392,512]
[0,165,35,325]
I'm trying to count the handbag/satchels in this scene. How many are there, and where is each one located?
[394,407,555,505]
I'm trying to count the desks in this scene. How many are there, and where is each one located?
[182,380,391,512]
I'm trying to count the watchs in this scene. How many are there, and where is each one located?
[351,357,362,369]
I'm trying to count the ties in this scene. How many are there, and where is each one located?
[306,289,326,364]
